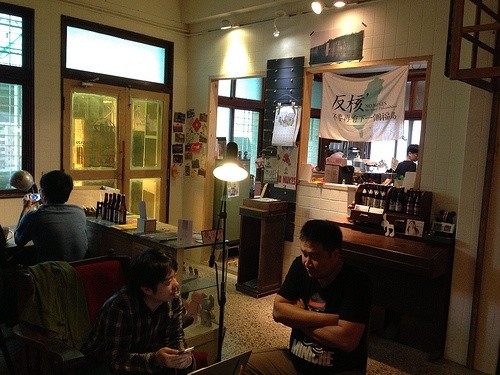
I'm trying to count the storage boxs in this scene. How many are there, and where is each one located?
[325,164,354,184]
[183,320,219,365]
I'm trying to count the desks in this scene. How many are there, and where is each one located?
[4,225,34,248]
[330,218,454,354]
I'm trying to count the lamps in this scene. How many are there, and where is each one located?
[311,0,346,14]
[207,141,249,362]
[9,170,38,194]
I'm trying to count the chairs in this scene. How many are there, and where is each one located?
[11,255,131,375]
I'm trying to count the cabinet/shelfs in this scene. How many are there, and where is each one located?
[86,213,229,368]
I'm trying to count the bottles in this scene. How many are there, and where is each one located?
[248,175,255,199]
[102,191,127,225]
[388,188,405,213]
[360,184,386,209]
[404,188,422,215]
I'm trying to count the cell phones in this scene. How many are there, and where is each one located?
[29,193,41,202]
[175,346,195,355]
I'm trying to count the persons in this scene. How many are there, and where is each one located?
[239,220,368,375]
[14,169,89,265]
[78,246,195,375]
[407,221,419,236]
[395,144,419,176]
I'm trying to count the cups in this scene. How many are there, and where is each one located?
[31,192,41,211]
